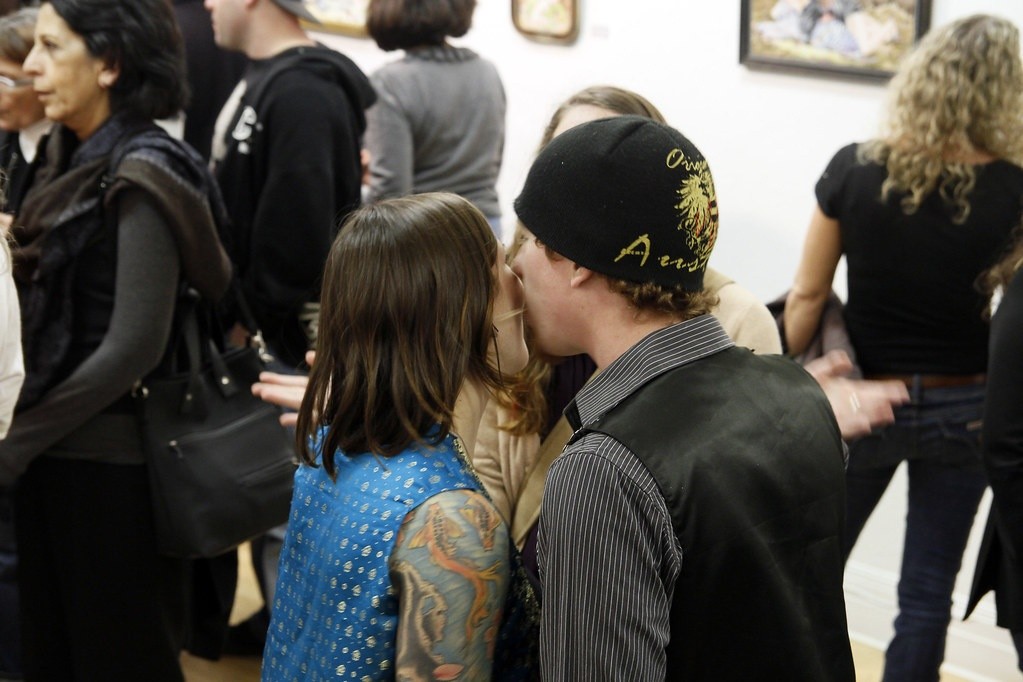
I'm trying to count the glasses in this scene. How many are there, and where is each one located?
[0,75,36,93]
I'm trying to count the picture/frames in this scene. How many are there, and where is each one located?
[512,0,578,44]
[739,0,933,83]
[298,0,373,39]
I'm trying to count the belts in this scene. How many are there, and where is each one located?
[874,371,986,389]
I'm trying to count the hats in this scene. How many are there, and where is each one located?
[514,114,719,286]
[275,0,318,23]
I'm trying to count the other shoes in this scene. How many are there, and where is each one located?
[229,610,272,655]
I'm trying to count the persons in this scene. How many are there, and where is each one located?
[781,16,1023,681]
[960,266,1023,669]
[260,190,539,682]
[0,0,914,682]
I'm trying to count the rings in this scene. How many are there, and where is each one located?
[850,394,859,410]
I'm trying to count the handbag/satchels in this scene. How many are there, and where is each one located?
[135,344,296,556]
